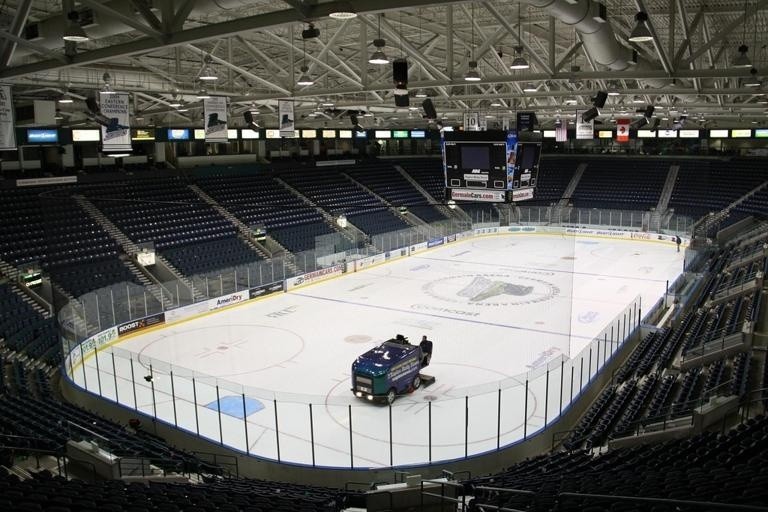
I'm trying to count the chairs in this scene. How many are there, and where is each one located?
[1,153,768,512]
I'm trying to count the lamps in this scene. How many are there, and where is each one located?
[53,0,762,130]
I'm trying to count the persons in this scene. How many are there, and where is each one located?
[675,235,682,252]
[420,335,433,365]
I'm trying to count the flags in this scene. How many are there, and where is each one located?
[616,118,630,142]
[554,118,568,143]
